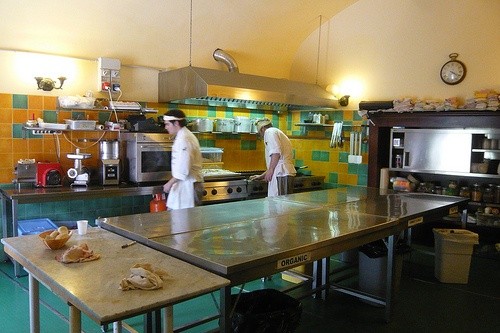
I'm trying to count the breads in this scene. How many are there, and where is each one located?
[48,226,69,240]
[485,207,499,214]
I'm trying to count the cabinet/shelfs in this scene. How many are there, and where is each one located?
[389,128,500,178]
[453,201,500,231]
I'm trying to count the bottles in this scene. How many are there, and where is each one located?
[482,134,500,149]
[308,112,325,124]
[395,154,402,168]
[418,180,500,205]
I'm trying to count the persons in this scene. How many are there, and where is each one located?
[255,119,297,197]
[161,109,207,208]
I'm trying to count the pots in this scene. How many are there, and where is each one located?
[233,116,252,132]
[294,166,308,170]
[214,118,235,132]
[251,118,260,132]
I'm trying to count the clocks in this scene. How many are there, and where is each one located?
[440,53,467,85]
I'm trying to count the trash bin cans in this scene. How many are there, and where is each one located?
[230,289,302,333]
[433,228,478,283]
[339,249,359,262]
[359,240,387,298]
[394,238,410,288]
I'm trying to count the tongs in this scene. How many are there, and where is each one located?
[330,123,344,149]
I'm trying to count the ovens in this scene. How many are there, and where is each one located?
[126,139,173,186]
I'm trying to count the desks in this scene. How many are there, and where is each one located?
[0,181,470,333]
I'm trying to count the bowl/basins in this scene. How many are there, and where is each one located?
[304,120,312,123]
[39,230,73,249]
[197,118,214,132]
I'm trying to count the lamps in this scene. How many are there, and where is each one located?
[35,76,66,91]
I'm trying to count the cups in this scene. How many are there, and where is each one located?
[380,168,389,189]
[77,220,88,234]
[471,163,488,174]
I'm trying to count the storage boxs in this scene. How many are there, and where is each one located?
[200,148,225,169]
[64,119,98,129]
[475,212,500,228]
[39,123,70,130]
[18,218,58,236]
[58,95,97,109]
[54,221,91,229]
